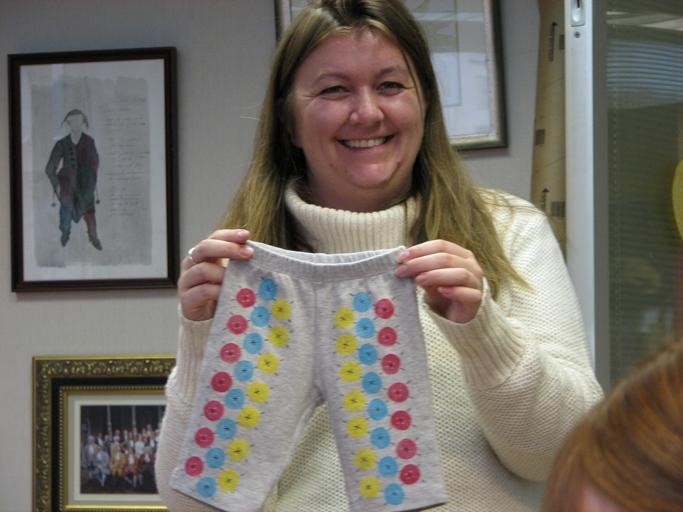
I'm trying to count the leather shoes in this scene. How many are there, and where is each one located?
[89,235,103,251]
[61,232,69,246]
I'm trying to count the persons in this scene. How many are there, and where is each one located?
[80,423,161,488]
[540,340,682,512]
[43,108,103,251]
[153,0,606,512]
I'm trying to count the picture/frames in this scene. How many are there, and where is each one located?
[30,353,178,510]
[7,45,181,293]
[274,0,509,156]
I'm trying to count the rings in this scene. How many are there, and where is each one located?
[187,247,202,264]
